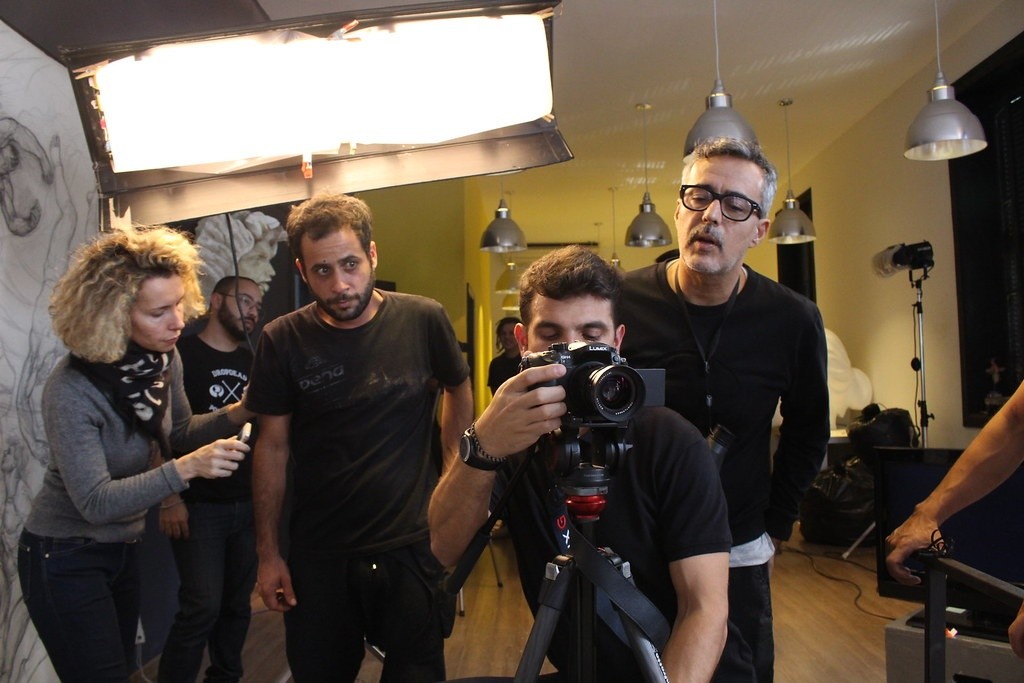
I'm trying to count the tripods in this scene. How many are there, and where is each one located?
[511,432,669,683]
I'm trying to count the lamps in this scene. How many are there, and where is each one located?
[683,0,760,154]
[502,290,519,312]
[903,1,988,164]
[492,258,524,293]
[766,98,816,245]
[607,185,627,278]
[624,103,673,248]
[480,173,527,256]
[63,0,575,232]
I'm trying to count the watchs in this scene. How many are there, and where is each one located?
[459,428,504,470]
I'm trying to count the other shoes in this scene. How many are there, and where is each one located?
[491,520,509,538]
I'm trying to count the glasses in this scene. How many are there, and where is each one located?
[218,292,263,314]
[679,185,762,222]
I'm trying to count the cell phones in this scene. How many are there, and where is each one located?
[236,422,252,443]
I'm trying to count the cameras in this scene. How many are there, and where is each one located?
[517,340,666,430]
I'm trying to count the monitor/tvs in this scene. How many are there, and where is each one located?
[872,445,1024,643]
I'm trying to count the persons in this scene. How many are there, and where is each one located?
[245,193,475,682]
[428,245,756,682]
[884,382,1024,659]
[18,225,257,683]
[487,318,521,396]
[154,276,261,683]
[621,137,831,683]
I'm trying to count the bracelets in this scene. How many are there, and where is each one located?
[471,422,507,461]
[161,500,184,509]
